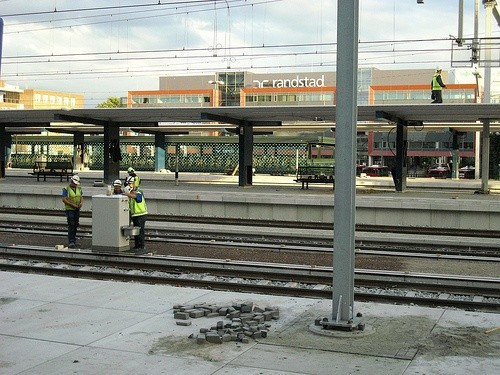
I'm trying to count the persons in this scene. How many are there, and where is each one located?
[124,167,142,192]
[33,154,39,174]
[62,175,83,248]
[122,184,148,251]
[429,66,447,103]
[111,179,124,195]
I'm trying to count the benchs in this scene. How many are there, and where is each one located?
[293,166,335,191]
[28,161,78,182]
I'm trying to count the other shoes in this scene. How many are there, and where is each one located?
[69,243,75,248]
[133,247,144,252]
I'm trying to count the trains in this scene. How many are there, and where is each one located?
[8,136,339,172]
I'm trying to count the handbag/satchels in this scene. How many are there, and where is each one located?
[431,91,435,100]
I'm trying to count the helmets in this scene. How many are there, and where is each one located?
[128,167,134,172]
[126,176,135,184]
[437,67,442,70]
[113,179,122,187]
[71,175,81,185]
